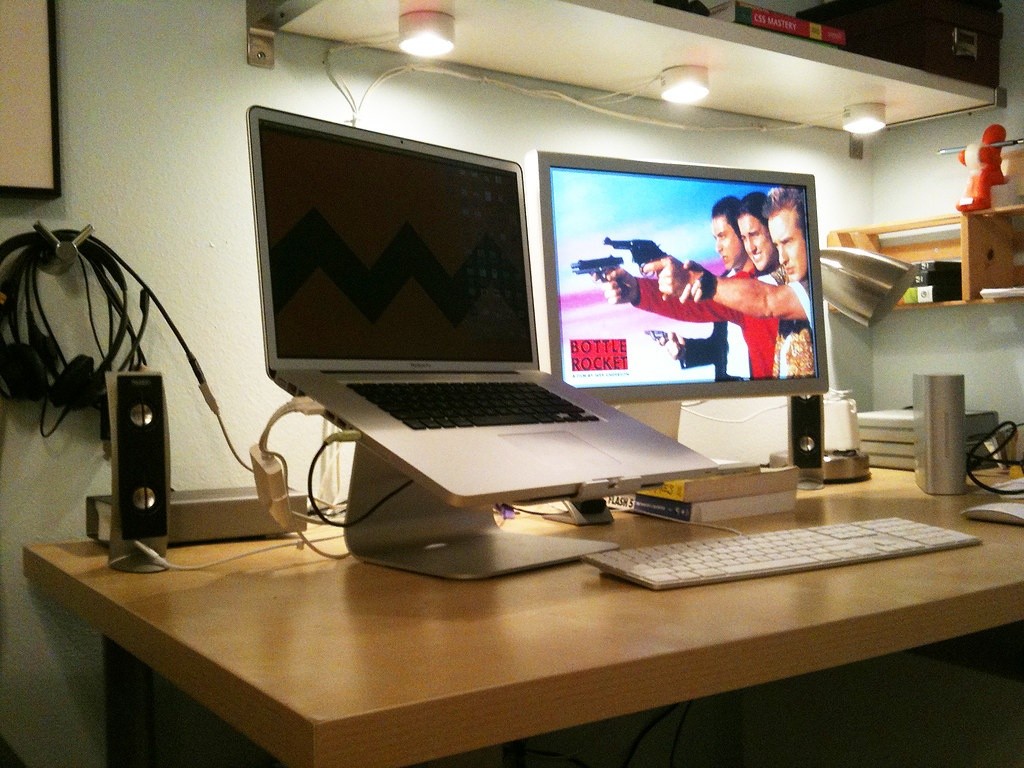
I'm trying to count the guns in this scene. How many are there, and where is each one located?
[572,255,623,282]
[603,236,666,274]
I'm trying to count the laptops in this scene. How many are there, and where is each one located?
[246,104,720,508]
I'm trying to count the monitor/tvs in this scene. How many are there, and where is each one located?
[522,149,830,406]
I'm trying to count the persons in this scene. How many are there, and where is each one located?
[591,187,816,382]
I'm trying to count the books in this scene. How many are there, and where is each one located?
[599,466,797,523]
[708,0,845,46]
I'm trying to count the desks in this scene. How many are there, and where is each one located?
[21,467,1024,768]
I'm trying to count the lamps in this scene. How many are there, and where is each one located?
[658,66,710,103]
[397,11,456,57]
[841,102,886,135]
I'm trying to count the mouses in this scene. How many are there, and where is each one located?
[960,502,1024,526]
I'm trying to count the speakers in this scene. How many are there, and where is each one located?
[787,394,824,490]
[105,371,171,573]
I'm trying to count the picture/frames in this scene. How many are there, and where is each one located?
[0,1,63,200]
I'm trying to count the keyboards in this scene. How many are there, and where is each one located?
[582,516,980,592]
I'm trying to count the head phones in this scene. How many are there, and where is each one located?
[0,229,149,410]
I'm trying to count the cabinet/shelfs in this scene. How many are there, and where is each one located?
[827,200,1024,314]
